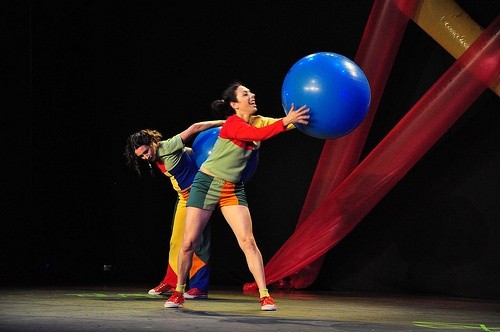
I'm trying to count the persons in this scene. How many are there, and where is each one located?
[164,83,310,312]
[126,120,226,299]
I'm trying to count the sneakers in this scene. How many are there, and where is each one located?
[164,291,185,307]
[183,287,208,299]
[257,295,277,310]
[148,282,171,295]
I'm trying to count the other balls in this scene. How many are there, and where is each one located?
[191,125,259,186]
[282,52,372,140]
